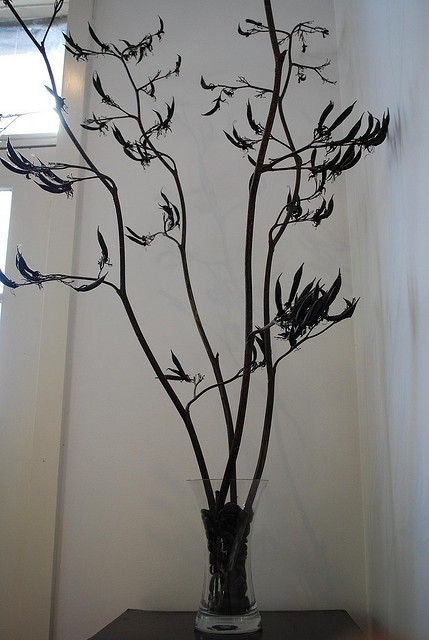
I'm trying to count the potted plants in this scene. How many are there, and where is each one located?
[2,0,390,635]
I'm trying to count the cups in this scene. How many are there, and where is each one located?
[186,478,270,634]
